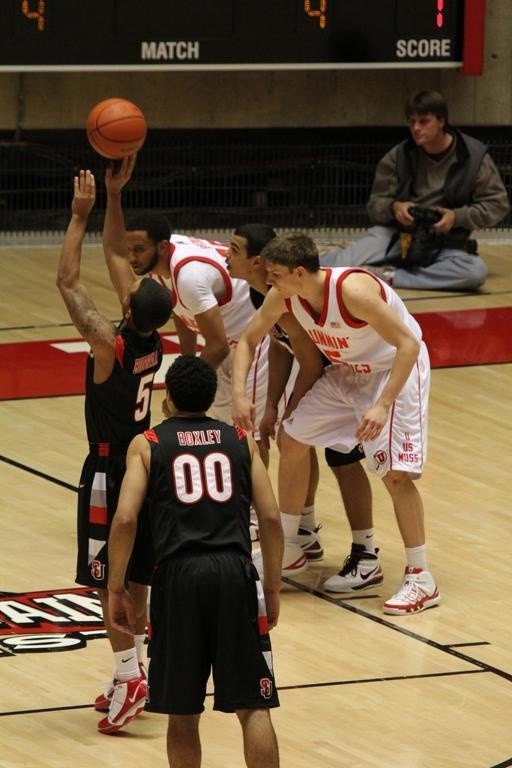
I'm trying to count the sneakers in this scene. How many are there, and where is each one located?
[382,566,440,615]
[252,543,308,576]
[98,677,150,734]
[95,662,148,710]
[322,544,383,593]
[316,242,345,257]
[297,524,324,561]
[364,266,396,288]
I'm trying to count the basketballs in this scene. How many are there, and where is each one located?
[87,98,147,158]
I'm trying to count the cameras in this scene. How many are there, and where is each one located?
[403,205,443,270]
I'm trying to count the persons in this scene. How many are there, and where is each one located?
[232,234,443,616]
[57,149,172,735]
[223,222,385,594]
[319,89,509,291]
[125,211,288,540]
[107,354,285,768]
